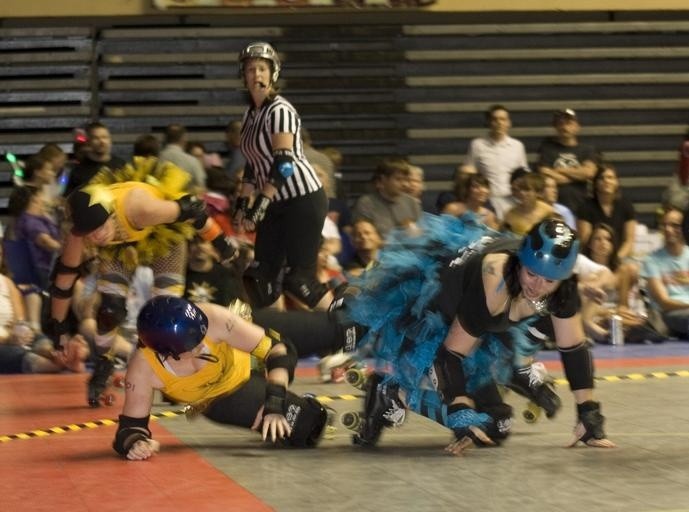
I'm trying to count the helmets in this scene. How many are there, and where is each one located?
[69,185,116,235]
[239,43,281,83]
[516,218,578,280]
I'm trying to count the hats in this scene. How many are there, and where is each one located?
[553,109,578,124]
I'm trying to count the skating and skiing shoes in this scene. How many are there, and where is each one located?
[341,362,405,444]
[302,392,337,440]
[87,351,124,407]
[494,362,560,422]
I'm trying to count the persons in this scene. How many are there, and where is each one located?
[360,211,616,455]
[113,282,370,463]
[230,42,353,375]
[2,127,338,375]
[341,108,689,351]
